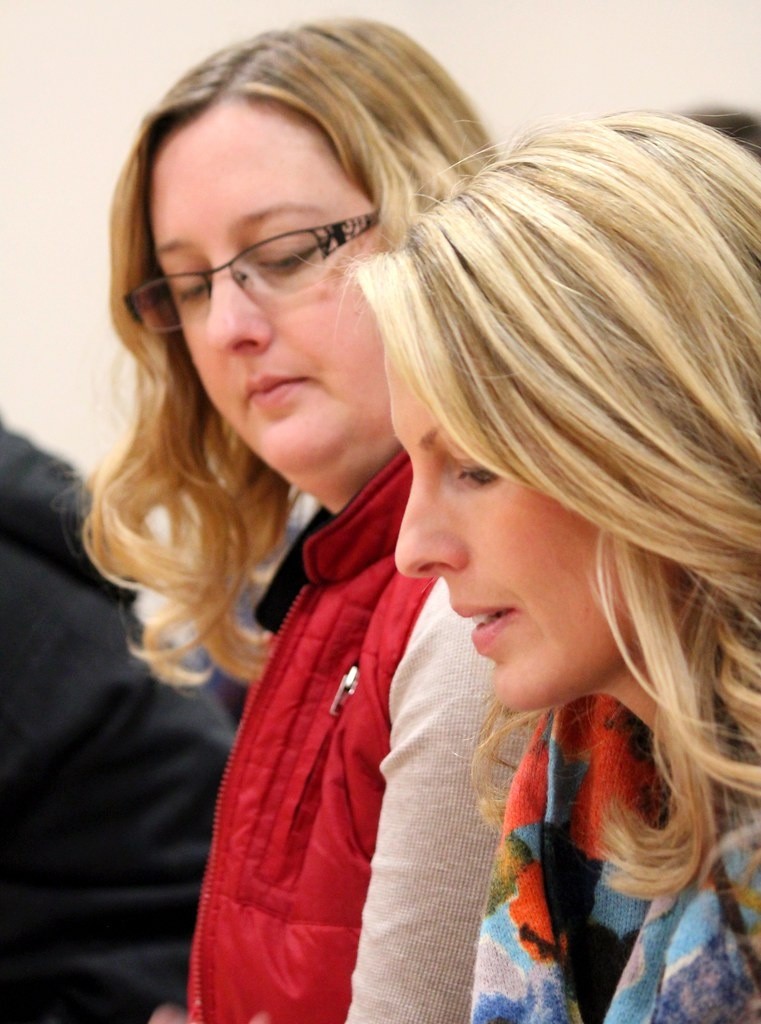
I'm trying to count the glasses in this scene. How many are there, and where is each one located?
[123,210,381,333]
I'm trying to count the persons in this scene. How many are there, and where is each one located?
[358,110,761,1024]
[82,17,540,1024]
[0,414,236,1024]
